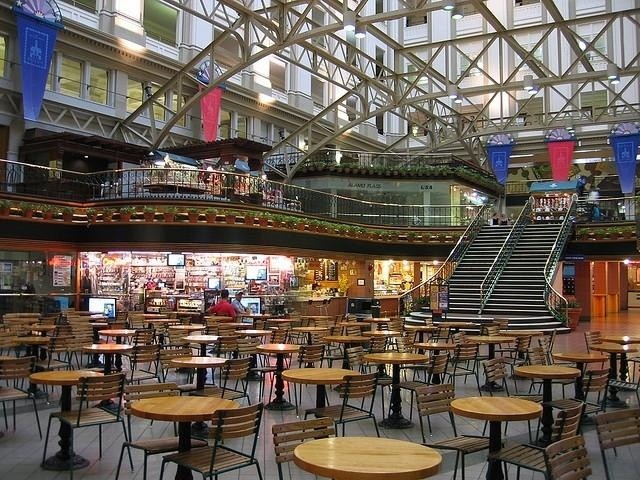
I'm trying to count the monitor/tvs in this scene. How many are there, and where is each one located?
[209,279,219,288]
[88,297,116,318]
[167,254,185,266]
[246,265,266,279]
[231,297,260,314]
[47,297,68,313]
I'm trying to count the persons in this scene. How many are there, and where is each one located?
[81,269,91,293]
[231,291,250,315]
[500,214,508,224]
[208,290,236,317]
[592,205,599,218]
[491,213,499,224]
